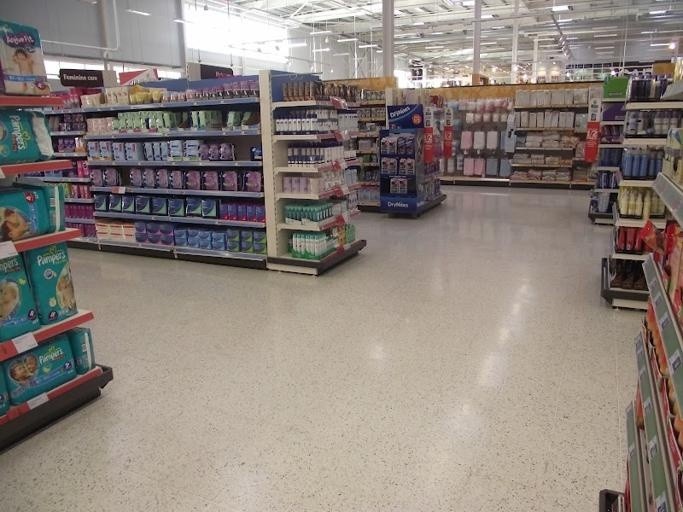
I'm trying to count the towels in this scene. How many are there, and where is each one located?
[458,130,511,177]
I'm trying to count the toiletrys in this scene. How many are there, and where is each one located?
[611,109,683,292]
[273,79,387,261]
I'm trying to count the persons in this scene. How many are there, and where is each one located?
[5,207,33,226]
[10,46,40,93]
[0,221,32,238]
[24,356,40,373]
[58,276,74,308]
[7,359,27,383]
[0,285,21,321]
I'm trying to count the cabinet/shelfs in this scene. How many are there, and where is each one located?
[269,99,366,276]
[334,101,384,209]
[599,101,682,312]
[0,91,113,447]
[80,92,265,272]
[586,96,626,227]
[39,107,98,253]
[601,129,683,512]
[507,102,598,186]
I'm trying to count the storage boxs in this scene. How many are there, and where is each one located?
[601,76,629,98]
